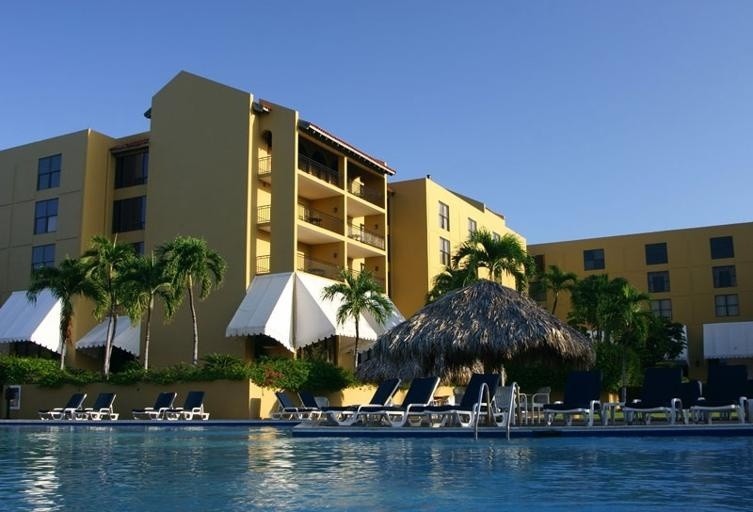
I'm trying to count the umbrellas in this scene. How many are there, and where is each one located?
[368,276,597,434]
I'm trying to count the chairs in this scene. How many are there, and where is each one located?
[357,376,440,426]
[691,369,749,425]
[623,369,681,425]
[165,390,210,421]
[682,382,702,423]
[618,386,643,405]
[37,393,87,421]
[424,374,502,428]
[64,392,120,420]
[473,382,517,427]
[131,391,177,420]
[314,397,329,408]
[322,377,401,425]
[543,370,600,426]
[271,392,321,420]
[297,389,358,423]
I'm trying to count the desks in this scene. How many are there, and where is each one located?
[306,217,323,226]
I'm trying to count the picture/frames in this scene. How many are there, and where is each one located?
[8,386,21,410]
